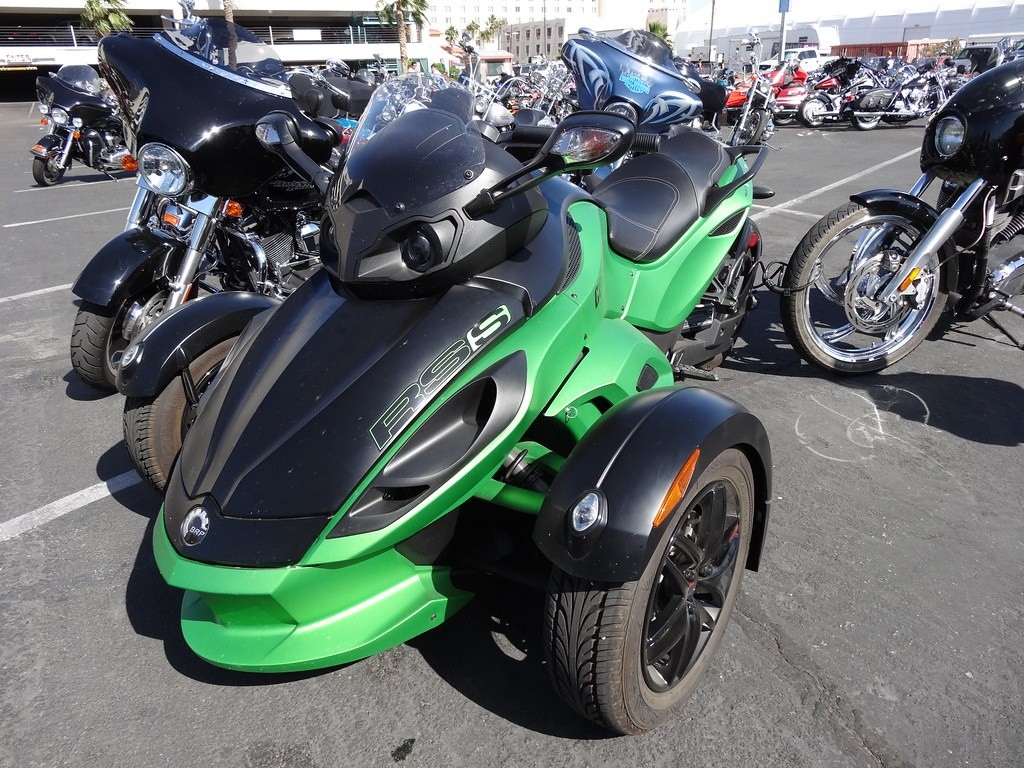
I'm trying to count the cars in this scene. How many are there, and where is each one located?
[1002,39,1024,65]
[950,37,1007,78]
[514,63,537,78]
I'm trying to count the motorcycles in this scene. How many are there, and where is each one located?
[28,0,1024,739]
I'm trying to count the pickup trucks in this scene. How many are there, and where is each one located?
[757,47,839,73]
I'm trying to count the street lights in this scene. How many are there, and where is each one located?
[504,32,519,54]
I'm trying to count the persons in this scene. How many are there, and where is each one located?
[431,64,442,75]
[407,61,420,73]
[696,58,705,59]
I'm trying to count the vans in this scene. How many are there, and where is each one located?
[534,60,567,81]
